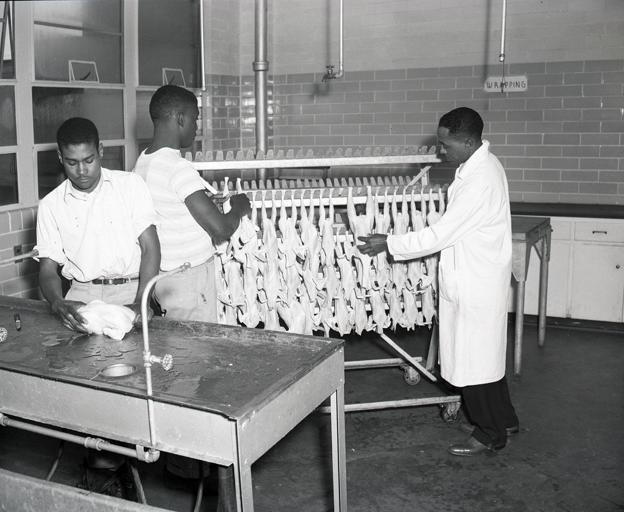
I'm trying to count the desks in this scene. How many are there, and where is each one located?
[506,214,554,381]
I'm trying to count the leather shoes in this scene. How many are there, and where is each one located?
[448,421,518,456]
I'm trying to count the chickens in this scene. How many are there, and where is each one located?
[213,176,446,338]
[62,299,138,340]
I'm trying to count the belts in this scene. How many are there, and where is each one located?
[92,278,126,284]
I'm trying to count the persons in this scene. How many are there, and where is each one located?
[127,84,252,320]
[32,116,160,333]
[355,106,520,457]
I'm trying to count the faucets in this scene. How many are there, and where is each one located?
[320,64,336,83]
[142,261,192,356]
